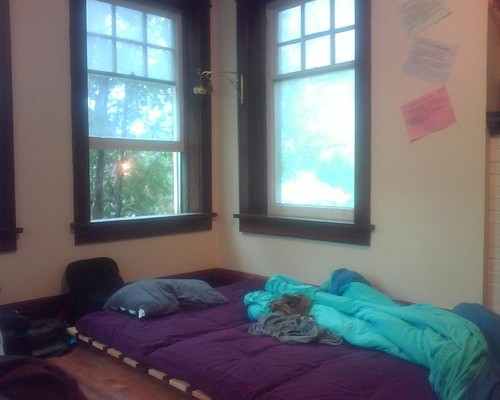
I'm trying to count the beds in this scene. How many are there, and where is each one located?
[67,267,499,399]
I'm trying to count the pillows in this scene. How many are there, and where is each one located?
[104,277,230,319]
